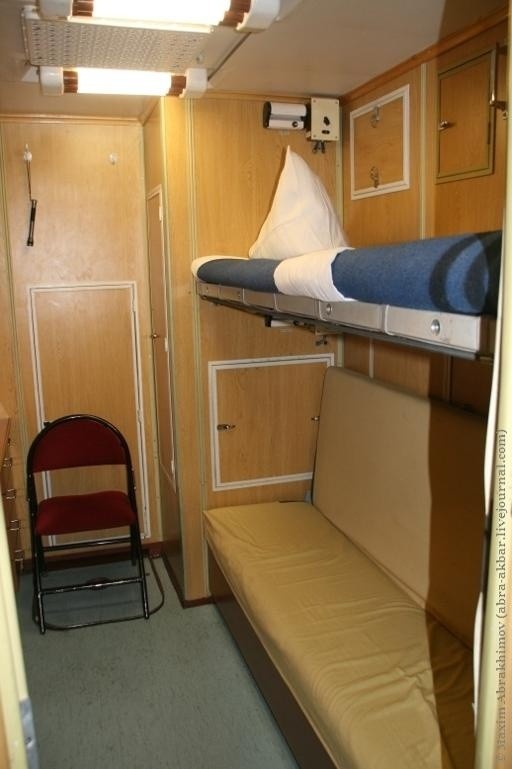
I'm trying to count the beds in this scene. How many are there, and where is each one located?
[196,272,495,366]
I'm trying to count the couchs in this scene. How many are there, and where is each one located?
[200,366,507,768]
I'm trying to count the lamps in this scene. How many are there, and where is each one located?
[35,66,209,101]
[48,0,279,41]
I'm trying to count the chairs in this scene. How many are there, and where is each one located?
[27,411,167,635]
